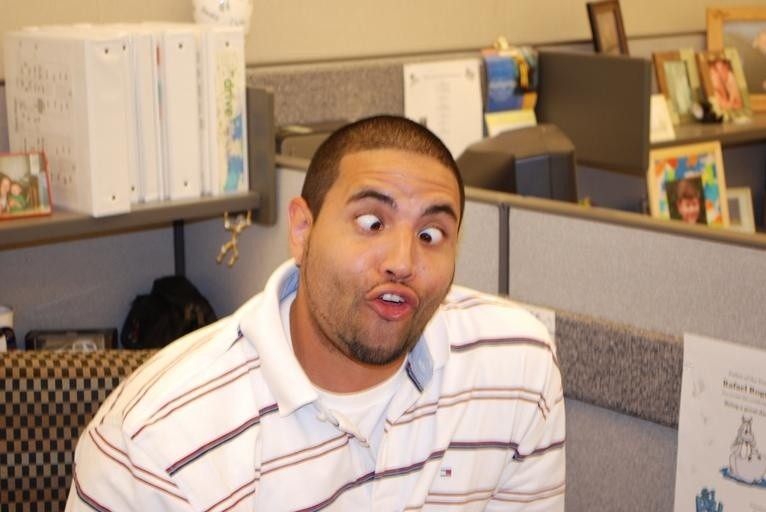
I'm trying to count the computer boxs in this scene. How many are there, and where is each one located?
[274,119,352,161]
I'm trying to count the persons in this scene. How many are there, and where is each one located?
[674,180,702,225]
[708,61,741,107]
[0,172,12,211]
[64,114,567,512]
[716,59,741,101]
[6,183,32,213]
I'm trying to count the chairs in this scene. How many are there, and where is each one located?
[0,348,171,512]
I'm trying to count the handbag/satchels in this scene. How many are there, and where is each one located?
[151,276,216,333]
[121,294,186,349]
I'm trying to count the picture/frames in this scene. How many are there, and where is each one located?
[0,150,53,221]
[652,47,708,126]
[586,0,629,55]
[696,46,753,123]
[24,327,120,351]
[647,141,732,227]
[726,186,756,235]
[706,7,766,114]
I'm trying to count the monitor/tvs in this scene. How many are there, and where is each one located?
[454,125,577,204]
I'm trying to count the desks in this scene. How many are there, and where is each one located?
[537,47,766,228]
